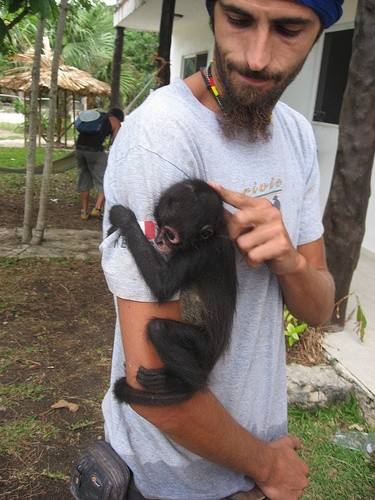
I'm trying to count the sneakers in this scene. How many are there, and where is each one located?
[80,209,91,219]
[90,207,104,218]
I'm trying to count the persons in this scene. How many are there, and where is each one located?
[75,108,125,219]
[98,0,344,500]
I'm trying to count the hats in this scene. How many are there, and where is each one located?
[206,0,343,29]
[110,108,124,122]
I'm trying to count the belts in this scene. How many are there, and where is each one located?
[76,147,102,151]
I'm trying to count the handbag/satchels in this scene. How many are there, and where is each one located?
[69,441,130,500]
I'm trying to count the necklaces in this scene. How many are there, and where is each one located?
[201,61,226,115]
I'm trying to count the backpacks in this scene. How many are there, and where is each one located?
[76,110,115,134]
